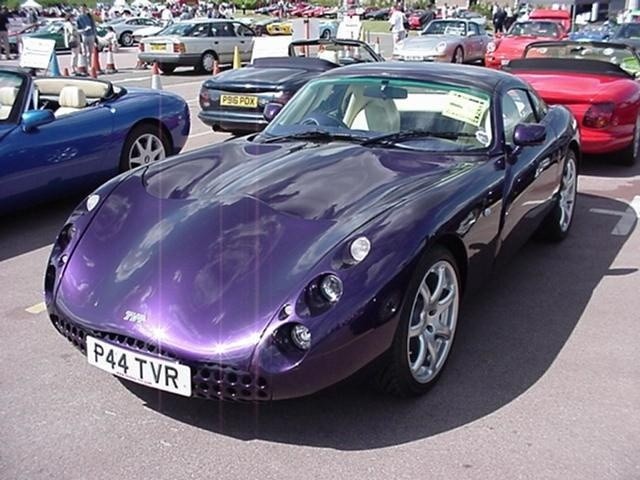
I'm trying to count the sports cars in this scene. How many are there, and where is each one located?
[198,40,385,135]
[0,65,191,213]
[498,40,639,166]
[393,19,488,64]
[45,59,582,404]
[484,8,572,69]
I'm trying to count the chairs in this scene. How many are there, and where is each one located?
[318,47,341,66]
[50,83,89,120]
[0,85,20,122]
[341,81,405,135]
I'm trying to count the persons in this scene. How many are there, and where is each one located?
[389,4,410,45]
[1,0,236,27]
[277,1,290,19]
[0,5,12,60]
[336,3,365,19]
[617,9,632,23]
[492,8,531,33]
[418,0,460,29]
[64,3,116,75]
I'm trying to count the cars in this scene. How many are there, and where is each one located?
[253,0,488,35]
[101,17,166,46]
[136,20,257,75]
[0,17,111,52]
[131,19,175,42]
[571,21,640,55]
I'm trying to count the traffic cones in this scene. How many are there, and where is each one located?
[299,26,382,61]
[135,56,147,69]
[212,59,220,75]
[46,48,60,76]
[63,42,118,77]
[150,61,164,91]
[232,46,243,69]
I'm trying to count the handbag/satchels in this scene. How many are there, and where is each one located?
[68,41,78,49]
[95,37,99,46]
